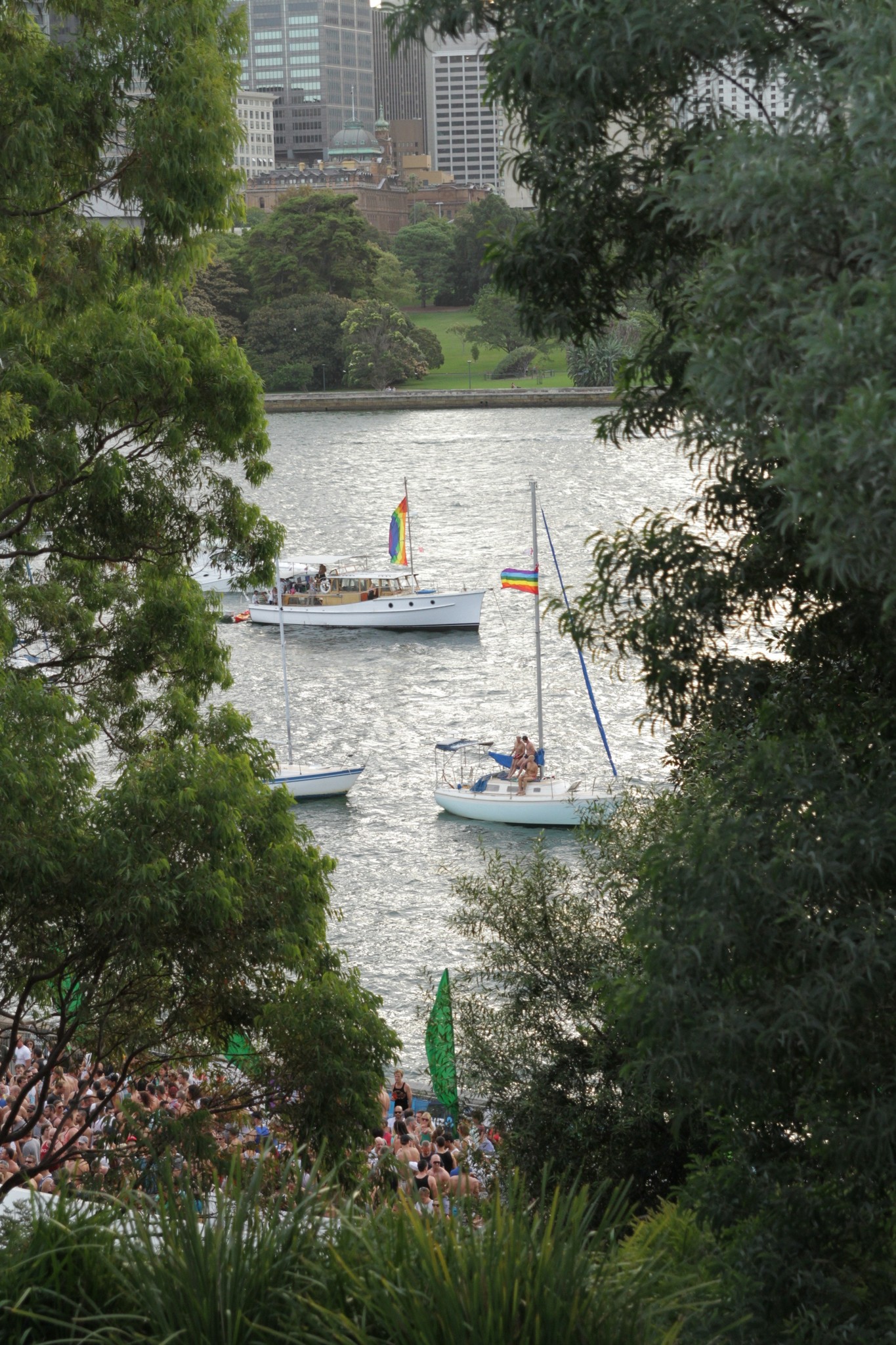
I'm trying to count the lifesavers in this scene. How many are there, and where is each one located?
[320,581,331,594]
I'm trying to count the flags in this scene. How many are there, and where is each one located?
[388,496,409,566]
[500,564,539,595]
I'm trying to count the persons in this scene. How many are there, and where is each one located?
[0,1034,519,1235]
[251,564,378,607]
[511,366,538,389]
[385,386,396,391]
[509,735,539,794]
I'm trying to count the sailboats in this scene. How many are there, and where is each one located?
[433,476,637,829]
[254,559,373,801]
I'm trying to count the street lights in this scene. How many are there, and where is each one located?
[322,364,326,390]
[467,361,472,389]
[436,202,444,218]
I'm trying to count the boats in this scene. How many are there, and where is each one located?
[249,563,485,632]
[191,549,320,594]
